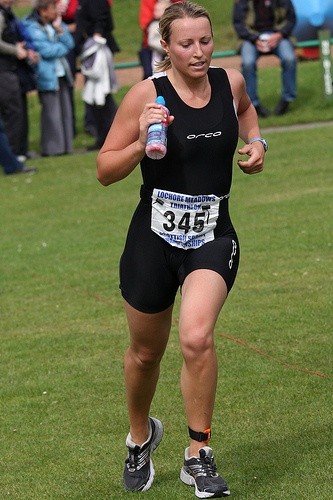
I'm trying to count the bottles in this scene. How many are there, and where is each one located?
[145,96,170,159]
[259,32,270,53]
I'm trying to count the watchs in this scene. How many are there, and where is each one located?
[250,137,268,152]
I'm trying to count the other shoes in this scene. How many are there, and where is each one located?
[20,166,38,172]
[275,101,288,115]
[254,105,269,117]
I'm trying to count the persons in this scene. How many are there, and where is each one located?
[0,0,39,175]
[96,1,265,499]
[139,0,183,80]
[233,0,297,117]
[20,0,75,157]
[51,0,120,150]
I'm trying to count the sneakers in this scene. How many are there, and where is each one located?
[178,444,230,499]
[123,416,163,491]
